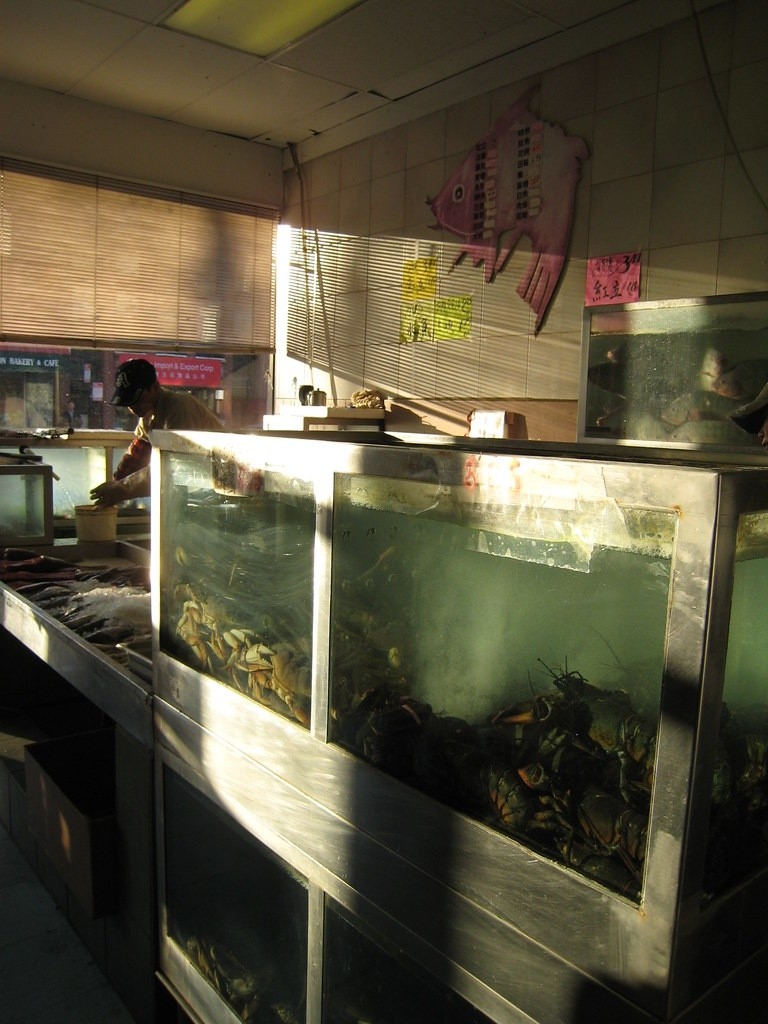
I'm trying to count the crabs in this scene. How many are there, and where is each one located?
[335,646,767,900]
[170,545,313,729]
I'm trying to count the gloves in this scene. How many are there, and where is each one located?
[91,466,150,512]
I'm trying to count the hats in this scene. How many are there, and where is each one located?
[107,359,155,407]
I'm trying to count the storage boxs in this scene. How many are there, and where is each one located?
[150,429,768,1024]
[24,723,116,918]
[578,289,768,454]
[0,435,150,549]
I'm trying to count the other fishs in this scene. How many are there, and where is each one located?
[587,332,768,450]
[424,81,591,336]
[0,548,151,645]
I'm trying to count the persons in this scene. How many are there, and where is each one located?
[90,358,227,510]
[56,402,83,429]
